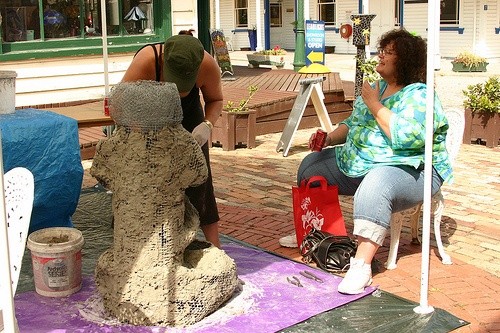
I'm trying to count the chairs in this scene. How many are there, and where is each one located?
[383,108,465,272]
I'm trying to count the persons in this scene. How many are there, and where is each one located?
[278,27,454,295]
[119,29,224,251]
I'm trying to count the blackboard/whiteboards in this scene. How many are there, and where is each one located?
[276,82,335,157]
[210,29,234,77]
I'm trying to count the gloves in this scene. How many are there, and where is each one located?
[192,122,211,148]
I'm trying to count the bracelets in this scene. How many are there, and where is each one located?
[203,119,214,130]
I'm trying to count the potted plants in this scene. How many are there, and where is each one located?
[451,51,489,72]
[247,48,287,70]
[206,85,260,151]
[462,74,500,148]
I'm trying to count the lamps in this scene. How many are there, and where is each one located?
[125,7,148,34]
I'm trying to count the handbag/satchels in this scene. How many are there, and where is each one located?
[292,176,347,253]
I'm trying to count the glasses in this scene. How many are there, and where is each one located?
[377,47,395,55]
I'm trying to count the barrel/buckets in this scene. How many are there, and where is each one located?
[27,226,86,298]
[0,70,18,114]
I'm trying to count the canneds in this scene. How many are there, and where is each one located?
[311,129,327,152]
[104,97,110,116]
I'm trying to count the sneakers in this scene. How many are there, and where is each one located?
[338,257,371,295]
[279,234,298,248]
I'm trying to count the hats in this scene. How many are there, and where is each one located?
[164,34,204,92]
